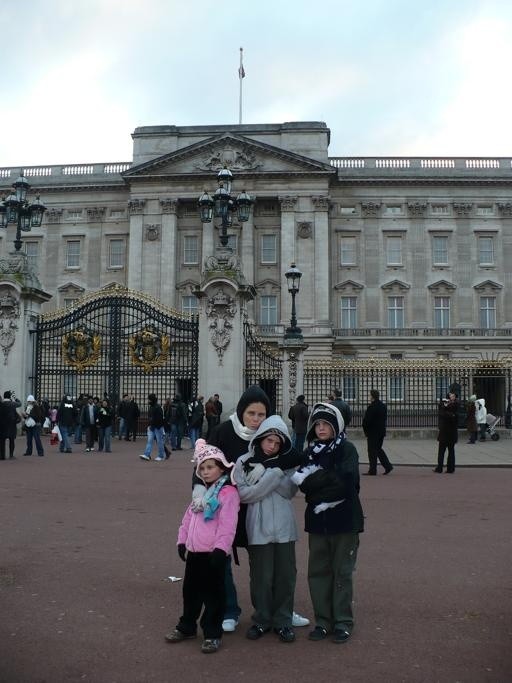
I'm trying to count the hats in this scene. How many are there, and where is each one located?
[469,394,476,401]
[309,405,339,440]
[147,393,156,401]
[193,438,234,482]
[252,427,286,453]
[27,395,35,401]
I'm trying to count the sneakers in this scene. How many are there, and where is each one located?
[1,455,16,460]
[431,468,455,473]
[308,625,328,642]
[467,438,485,444]
[23,452,44,456]
[59,447,73,453]
[165,629,199,643]
[246,622,271,640]
[221,617,238,631]
[201,638,222,654]
[361,465,393,476]
[331,627,353,644]
[171,447,183,451]
[275,627,296,642]
[85,447,112,453]
[139,450,171,461]
[292,610,310,627]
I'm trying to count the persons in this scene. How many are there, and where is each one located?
[288,394,309,451]
[230,415,299,643]
[464,394,478,444]
[362,389,393,475]
[291,401,364,644]
[475,399,487,442]
[329,388,353,433]
[164,438,241,654]
[447,391,457,416]
[191,386,310,632]
[0,391,222,462]
[431,402,459,473]
[328,396,334,403]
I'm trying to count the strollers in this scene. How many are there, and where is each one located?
[468,413,502,441]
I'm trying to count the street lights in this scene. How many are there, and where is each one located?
[370,356,375,390]
[439,356,444,402]
[0,171,48,255]
[198,165,253,253]
[283,260,304,339]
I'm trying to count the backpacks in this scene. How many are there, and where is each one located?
[169,402,182,420]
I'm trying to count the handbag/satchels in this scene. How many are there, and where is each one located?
[50,425,62,445]
[22,417,36,434]
